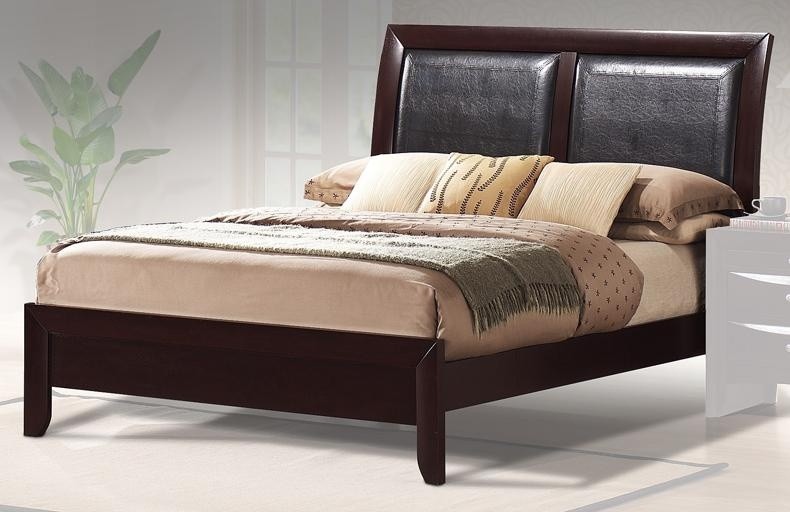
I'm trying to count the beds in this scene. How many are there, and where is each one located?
[23,25,774,485]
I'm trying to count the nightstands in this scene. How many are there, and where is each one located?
[706,226,788,417]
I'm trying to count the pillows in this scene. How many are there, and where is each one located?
[618,163,744,231]
[340,152,449,213]
[518,162,643,238]
[304,156,373,208]
[417,152,553,220]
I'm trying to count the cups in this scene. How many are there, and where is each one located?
[751,196,787,215]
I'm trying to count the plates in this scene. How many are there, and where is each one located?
[749,211,790,219]
